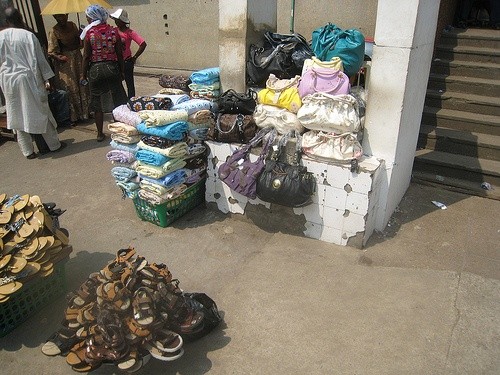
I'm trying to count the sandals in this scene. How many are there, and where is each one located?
[41,247,204,375]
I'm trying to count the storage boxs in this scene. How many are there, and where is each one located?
[0,256,70,339]
[134,174,206,228]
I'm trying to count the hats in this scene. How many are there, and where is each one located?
[110,8,130,24]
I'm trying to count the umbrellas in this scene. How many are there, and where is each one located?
[40,0,113,46]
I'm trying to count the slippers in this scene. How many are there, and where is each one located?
[0,193,73,304]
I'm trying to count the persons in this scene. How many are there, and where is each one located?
[0,6,67,159]
[46,5,147,142]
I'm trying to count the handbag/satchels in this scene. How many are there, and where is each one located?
[214,21,366,209]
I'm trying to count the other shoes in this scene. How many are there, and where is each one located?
[51,141,67,152]
[96,133,105,142]
[26,152,36,159]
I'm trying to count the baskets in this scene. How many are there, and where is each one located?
[0,256,69,340]
[131,178,206,228]
